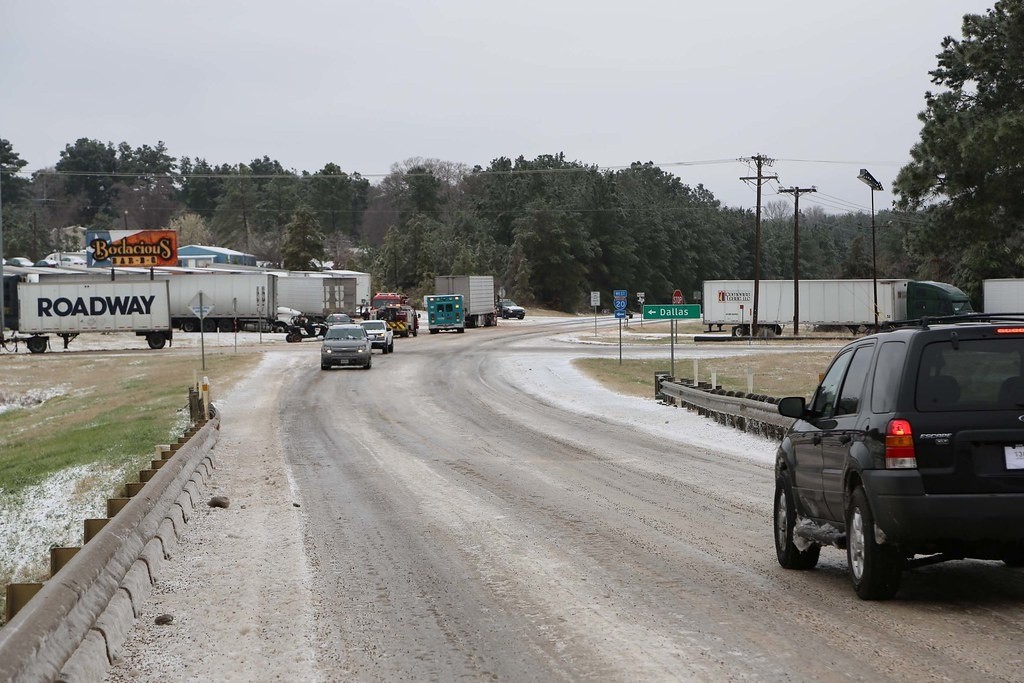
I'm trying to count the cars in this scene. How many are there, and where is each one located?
[286,313,329,343]
[320,325,376,370]
[323,313,356,330]
[357,320,394,354]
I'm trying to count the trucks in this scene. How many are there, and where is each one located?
[369,292,421,339]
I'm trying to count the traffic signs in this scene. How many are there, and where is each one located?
[642,304,700,319]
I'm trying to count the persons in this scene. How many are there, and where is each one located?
[362,308,370,320]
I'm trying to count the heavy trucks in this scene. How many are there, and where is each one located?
[702,279,974,336]
[435,275,497,328]
[0,251,303,353]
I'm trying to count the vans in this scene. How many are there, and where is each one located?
[426,295,465,334]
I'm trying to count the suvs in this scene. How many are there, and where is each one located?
[494,299,525,320]
[772,311,1024,601]
